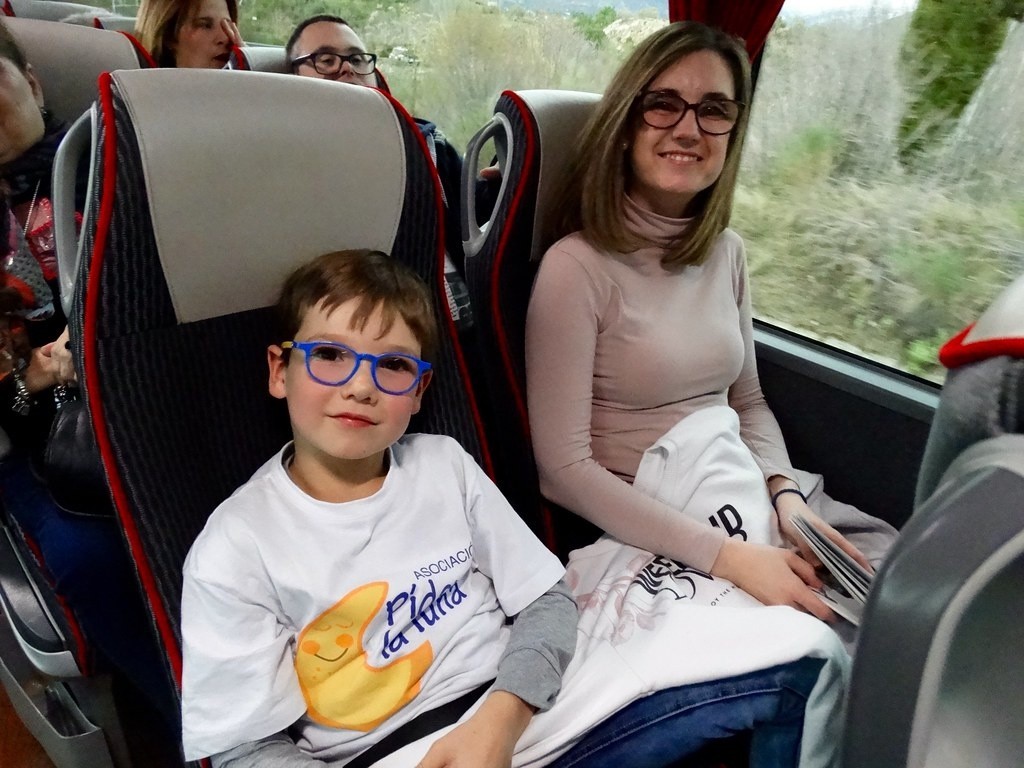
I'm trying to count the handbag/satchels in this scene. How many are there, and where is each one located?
[41,378,119,522]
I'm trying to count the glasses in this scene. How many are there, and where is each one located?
[278,340,427,396]
[290,45,381,76]
[631,93,744,135]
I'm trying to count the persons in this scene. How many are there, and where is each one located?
[282,15,462,250]
[181,248,850,768]
[525,19,851,626]
[0,20,196,768]
[136,1,246,70]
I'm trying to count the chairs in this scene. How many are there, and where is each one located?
[459,87,603,565]
[0,16,158,120]
[221,45,391,95]
[52,66,494,768]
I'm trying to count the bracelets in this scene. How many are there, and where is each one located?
[772,489,807,511]
